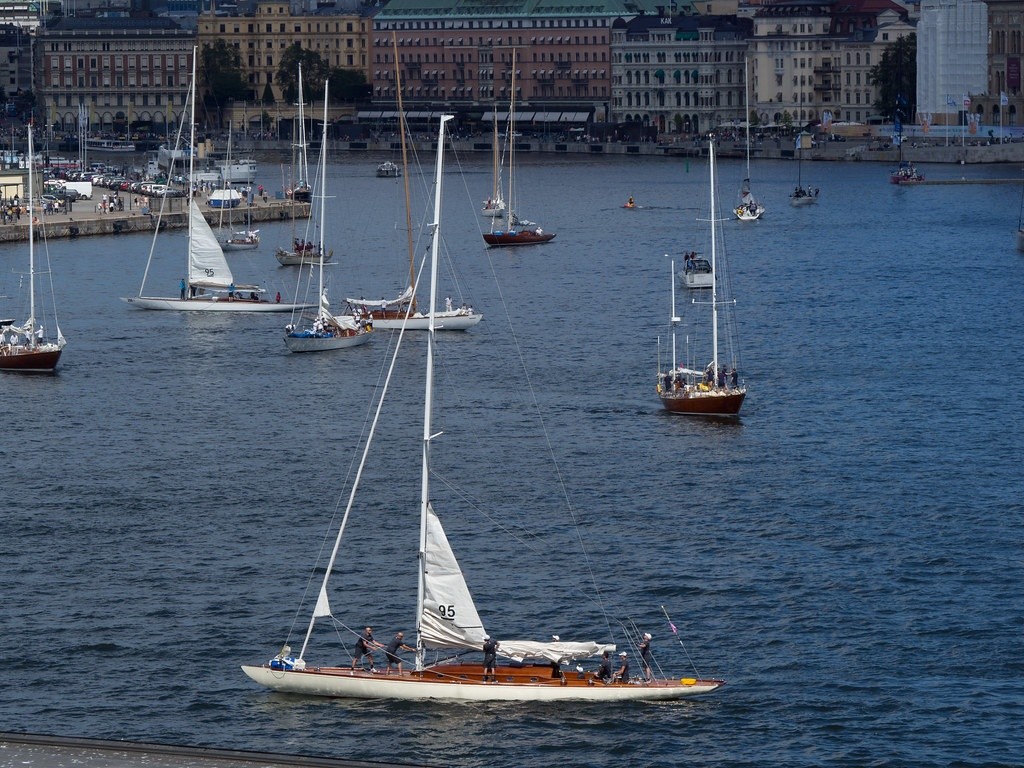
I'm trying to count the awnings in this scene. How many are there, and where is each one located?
[358,111,445,118]
[482,112,589,122]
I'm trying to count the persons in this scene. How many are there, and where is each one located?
[894,164,921,182]
[550,633,560,677]
[445,295,474,315]
[354,306,374,331]
[361,296,367,312]
[228,282,237,300]
[381,297,388,319]
[384,632,416,678]
[594,651,612,682]
[535,227,545,235]
[350,627,384,675]
[397,290,404,312]
[276,292,282,303]
[640,631,653,682]
[481,635,500,684]
[0,325,45,346]
[0,150,270,226]
[313,316,329,332]
[664,364,739,390]
[179,278,186,299]
[611,651,629,683]
[812,128,1024,150]
[0,120,798,148]
[807,184,819,197]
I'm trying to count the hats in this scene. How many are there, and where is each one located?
[618,651,627,656]
[482,635,490,640]
[644,632,652,640]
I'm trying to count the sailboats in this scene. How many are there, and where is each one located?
[157,58,341,266]
[474,45,560,248]
[479,103,506,217]
[890,42,928,184]
[336,28,490,332]
[789,72,820,207]
[119,46,321,314]
[236,113,727,707]
[0,123,66,373]
[659,130,751,422]
[732,56,767,222]
[273,82,377,354]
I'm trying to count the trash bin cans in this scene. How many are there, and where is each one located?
[247,194,254,206]
[65,202,72,211]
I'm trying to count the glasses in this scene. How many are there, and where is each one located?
[398,636,403,638]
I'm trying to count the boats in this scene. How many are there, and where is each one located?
[678,257,714,290]
[623,203,638,209]
[0,319,15,329]
[374,160,404,180]
[81,103,136,152]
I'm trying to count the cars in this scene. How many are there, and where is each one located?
[41,163,156,196]
[41,194,66,206]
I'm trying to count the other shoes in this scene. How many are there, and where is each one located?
[482,678,487,683]
[492,680,498,684]
[350,669,356,672]
[370,668,377,673]
[644,679,652,683]
